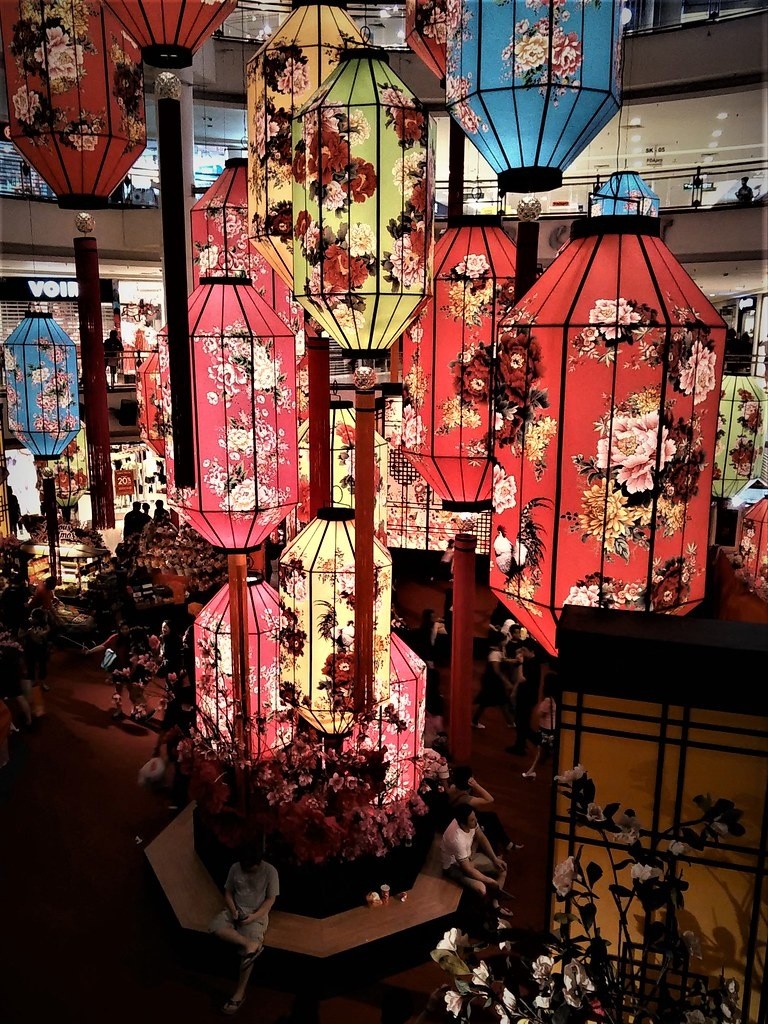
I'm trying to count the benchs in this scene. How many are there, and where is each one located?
[143,799,466,960]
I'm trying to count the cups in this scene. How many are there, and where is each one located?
[380,885,390,906]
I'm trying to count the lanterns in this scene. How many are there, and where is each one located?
[741,496,768,593]
[0,0,239,531]
[486,220,726,664]
[135,0,433,761]
[196,506,425,805]
[402,2,660,305]
[398,218,546,758]
[3,311,80,575]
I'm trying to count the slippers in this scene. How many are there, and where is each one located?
[221,994,247,1014]
[240,946,264,972]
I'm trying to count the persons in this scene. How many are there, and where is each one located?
[85,618,195,756]
[723,327,753,373]
[7,486,21,537]
[124,500,169,541]
[0,574,32,640]
[25,576,68,622]
[432,538,455,585]
[440,804,513,930]
[209,848,280,1013]
[521,674,556,781]
[0,624,33,805]
[19,610,51,691]
[103,330,124,390]
[443,766,523,862]
[470,624,542,754]
[409,608,448,718]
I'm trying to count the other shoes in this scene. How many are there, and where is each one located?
[9,726,20,735]
[111,711,122,719]
[494,906,514,917]
[42,685,51,691]
[471,722,485,729]
[142,710,155,722]
[505,746,527,756]
[508,722,516,728]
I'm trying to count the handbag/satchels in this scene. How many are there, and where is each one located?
[541,732,553,746]
[100,648,117,669]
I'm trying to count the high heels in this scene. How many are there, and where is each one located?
[522,771,536,781]
